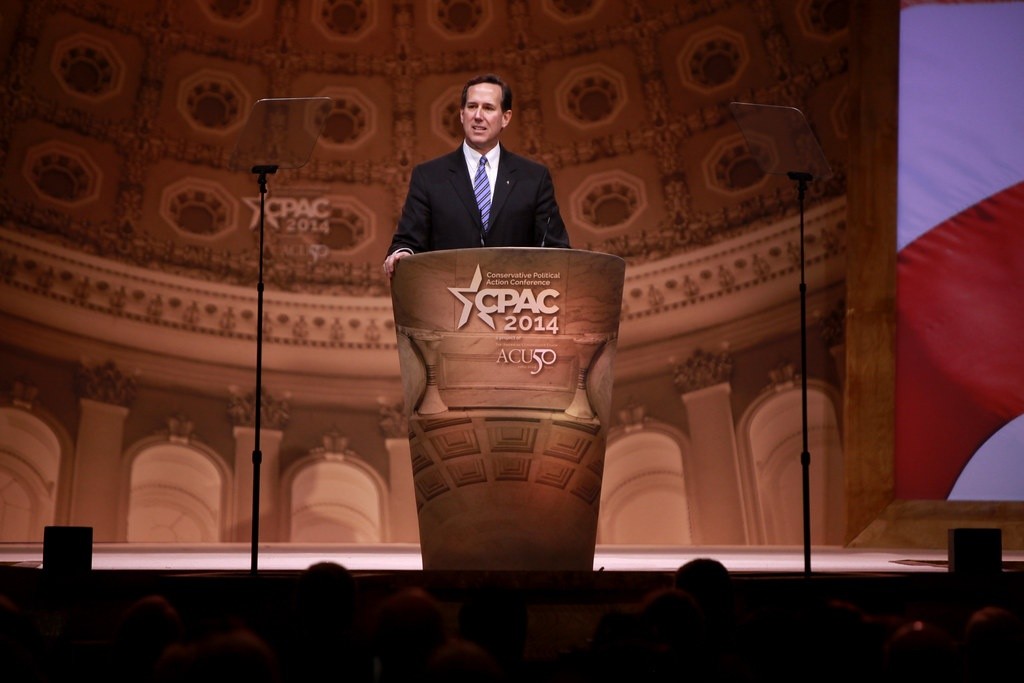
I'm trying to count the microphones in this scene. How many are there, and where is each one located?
[479,209,485,247]
[541,209,553,247]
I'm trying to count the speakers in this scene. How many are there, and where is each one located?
[948,528,1002,573]
[42,525,93,571]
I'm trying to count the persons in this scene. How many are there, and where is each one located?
[0,559,1024,683]
[380,75,572,277]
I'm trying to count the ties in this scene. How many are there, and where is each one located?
[473,156,491,235]
